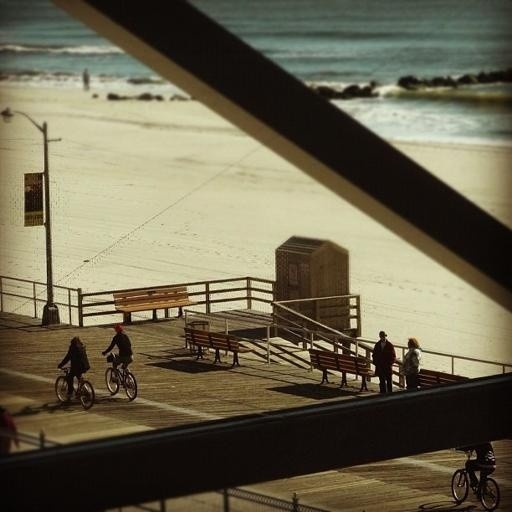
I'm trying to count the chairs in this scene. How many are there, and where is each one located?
[189,320,209,354]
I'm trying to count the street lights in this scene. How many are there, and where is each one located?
[1,108,59,327]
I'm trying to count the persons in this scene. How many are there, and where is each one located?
[101,324,133,383]
[56,334,89,401]
[0,403,21,454]
[455,442,496,496]
[400,337,420,389]
[372,330,395,395]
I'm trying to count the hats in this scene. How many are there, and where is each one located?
[408,337,419,348]
[115,324,124,333]
[379,331,387,336]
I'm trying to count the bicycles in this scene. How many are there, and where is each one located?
[450,449,502,511]
[56,366,96,410]
[105,352,139,401]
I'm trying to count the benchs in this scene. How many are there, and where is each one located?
[309,349,376,392]
[182,327,255,367]
[418,369,469,389]
[113,285,192,324]
